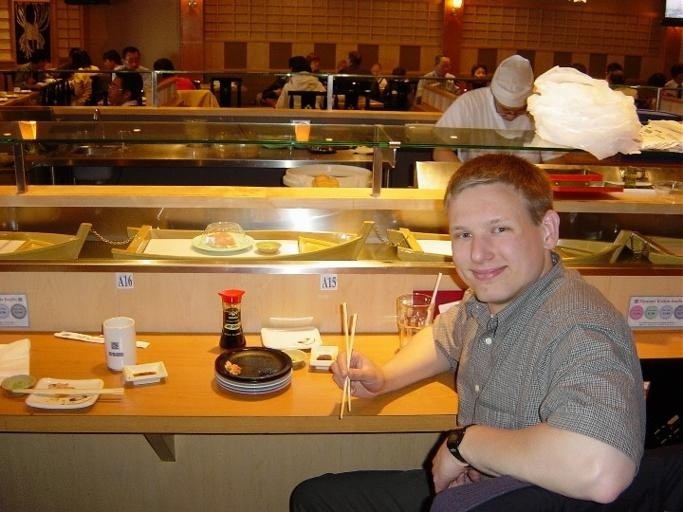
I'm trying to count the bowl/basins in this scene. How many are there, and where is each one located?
[654,179,682,201]
[13,87,21,93]
[201,218,246,252]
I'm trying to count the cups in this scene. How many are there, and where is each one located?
[104,317,137,373]
[398,295,435,355]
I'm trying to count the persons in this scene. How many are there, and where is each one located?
[153,58,196,90]
[112,46,153,91]
[263,74,291,109]
[308,59,327,85]
[335,60,348,73]
[460,64,487,92]
[65,48,101,107]
[414,56,451,104]
[605,70,638,104]
[383,67,414,108]
[102,73,146,110]
[605,63,624,82]
[334,51,381,100]
[99,51,123,72]
[432,54,536,162]
[289,153,647,511]
[666,65,683,100]
[13,48,55,90]
[370,64,387,93]
[276,56,327,110]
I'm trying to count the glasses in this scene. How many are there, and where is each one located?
[107,83,125,93]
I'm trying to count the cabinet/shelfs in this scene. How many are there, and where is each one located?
[0,121,683,334]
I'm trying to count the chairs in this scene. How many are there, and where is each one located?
[289,90,328,110]
[209,76,244,107]
[83,91,108,106]
[333,82,372,109]
[41,80,71,104]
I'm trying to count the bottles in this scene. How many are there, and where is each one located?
[217,290,246,349]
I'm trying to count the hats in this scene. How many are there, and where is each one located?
[490,56,536,110]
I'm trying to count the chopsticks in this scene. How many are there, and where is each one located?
[421,272,446,330]
[11,385,125,396]
[340,302,358,421]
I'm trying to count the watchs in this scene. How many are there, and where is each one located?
[447,423,476,463]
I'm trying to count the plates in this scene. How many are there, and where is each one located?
[257,240,281,255]
[0,375,36,397]
[191,233,255,255]
[311,345,339,370]
[5,93,20,98]
[20,90,31,95]
[215,348,293,382]
[284,350,311,369]
[257,327,326,350]
[308,348,336,374]
[1,96,8,103]
[207,375,295,396]
[26,377,103,408]
[125,362,169,389]
